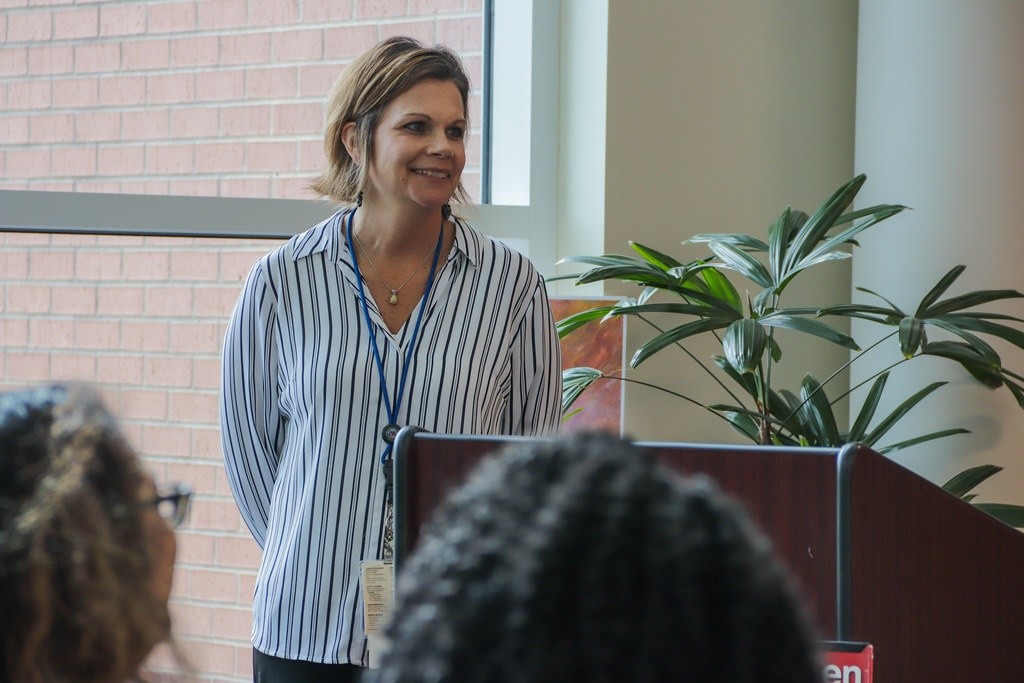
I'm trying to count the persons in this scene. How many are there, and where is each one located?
[219,38,562,683]
[362,426,829,683]
[0,380,194,683]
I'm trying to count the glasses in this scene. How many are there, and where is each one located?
[109,481,193,533]
